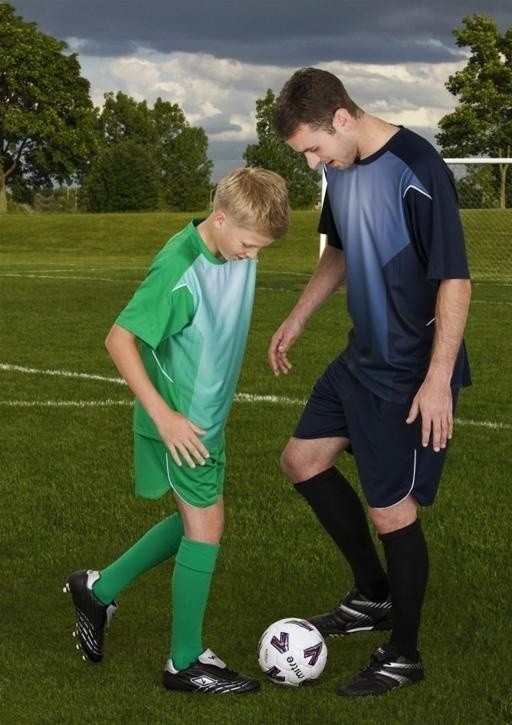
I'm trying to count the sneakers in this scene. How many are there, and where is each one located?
[306,583,395,636]
[161,647,259,692]
[335,645,426,697]
[65,568,117,661]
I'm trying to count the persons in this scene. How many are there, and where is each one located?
[262,62,476,700]
[63,166,291,698]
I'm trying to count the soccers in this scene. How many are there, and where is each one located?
[257,617,328,689]
